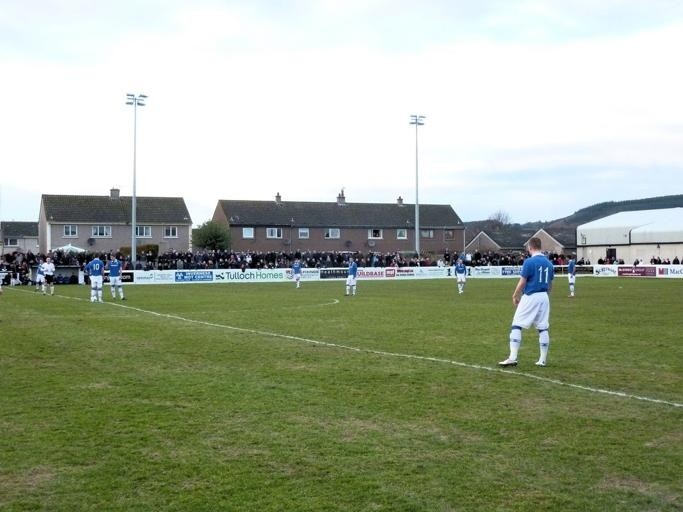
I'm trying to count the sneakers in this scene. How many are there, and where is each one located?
[497,358,518,367]
[534,361,547,367]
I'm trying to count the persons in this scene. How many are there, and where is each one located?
[499,237,554,366]
[454,258,466,295]
[83,252,105,303]
[35,256,55,296]
[344,256,358,296]
[598,256,624,265]
[1,248,134,286]
[104,254,125,300]
[633,255,682,266]
[135,248,590,272]
[565,255,576,297]
[291,258,302,288]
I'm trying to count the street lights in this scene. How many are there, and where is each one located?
[409,114,426,262]
[125,92,148,270]
